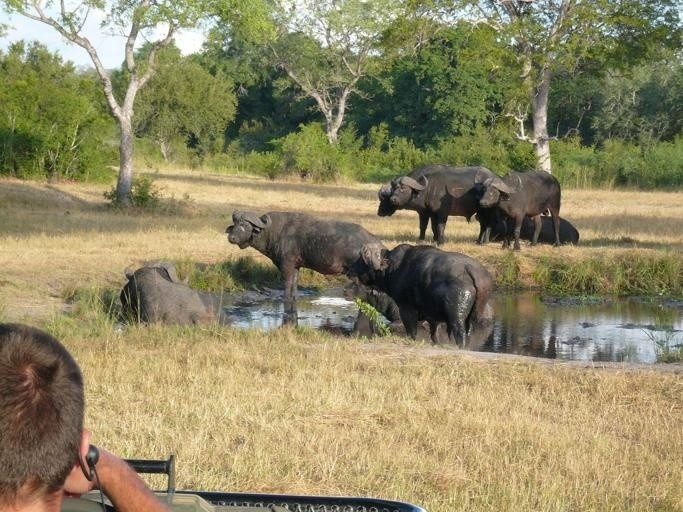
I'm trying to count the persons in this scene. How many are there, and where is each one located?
[1,321,176,512]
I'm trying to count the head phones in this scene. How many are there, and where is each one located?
[85,443,100,466]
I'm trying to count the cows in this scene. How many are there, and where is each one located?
[341,243,493,352]
[223,209,387,299]
[376,163,579,252]
[118,261,228,327]
